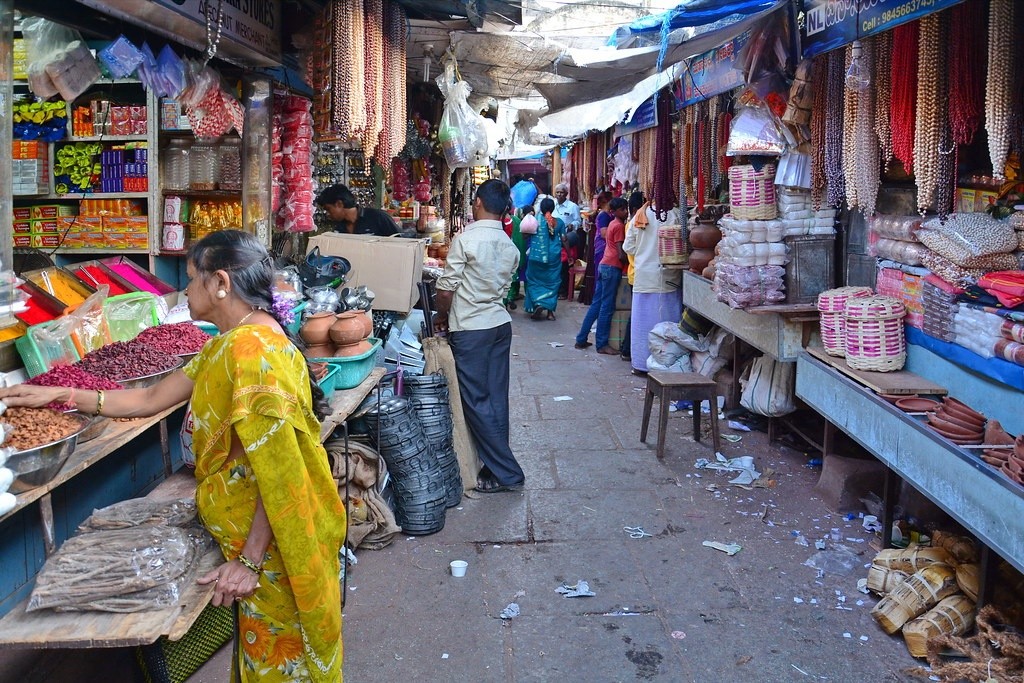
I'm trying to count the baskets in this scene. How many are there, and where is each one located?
[14,291,160,378]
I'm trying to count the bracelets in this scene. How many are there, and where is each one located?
[66,387,75,407]
[238,554,261,574]
[93,390,104,416]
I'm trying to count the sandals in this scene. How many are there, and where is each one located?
[475,478,524,493]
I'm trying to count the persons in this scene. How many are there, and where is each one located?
[622,199,690,375]
[499,177,582,320]
[0,229,346,683]
[316,184,408,238]
[575,191,648,360]
[433,179,525,493]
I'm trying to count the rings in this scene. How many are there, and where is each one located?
[235,596,242,601]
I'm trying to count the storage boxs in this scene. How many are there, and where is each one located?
[306,232,427,313]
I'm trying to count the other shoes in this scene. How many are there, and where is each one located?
[508,300,517,310]
[622,355,630,361]
[516,294,524,300]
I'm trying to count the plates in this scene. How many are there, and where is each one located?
[877,391,1024,487]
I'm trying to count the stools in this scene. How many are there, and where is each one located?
[640,371,721,462]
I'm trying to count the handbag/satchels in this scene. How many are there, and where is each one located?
[740,353,798,418]
[519,213,539,234]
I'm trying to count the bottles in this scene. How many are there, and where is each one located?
[300,310,373,358]
[688,219,722,279]
[163,134,241,191]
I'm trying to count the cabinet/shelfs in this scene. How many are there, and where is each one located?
[12,77,240,290]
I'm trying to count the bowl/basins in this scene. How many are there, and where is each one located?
[176,352,199,367]
[4,414,91,494]
[63,409,111,444]
[116,357,184,389]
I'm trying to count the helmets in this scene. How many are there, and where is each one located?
[296,246,351,289]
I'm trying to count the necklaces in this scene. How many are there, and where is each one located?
[216,307,255,339]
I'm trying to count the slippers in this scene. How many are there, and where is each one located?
[546,315,555,321]
[531,306,542,319]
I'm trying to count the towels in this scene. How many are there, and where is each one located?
[634,201,650,230]
